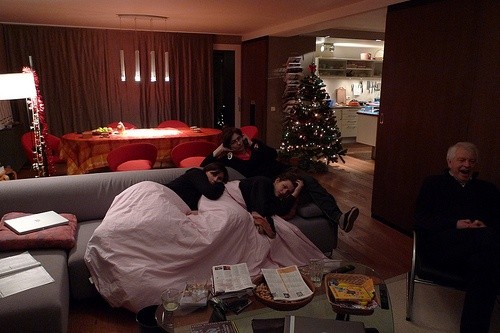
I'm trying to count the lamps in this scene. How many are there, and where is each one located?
[0,72,37,101]
[118,13,170,82]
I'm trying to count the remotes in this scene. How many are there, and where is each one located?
[323,264,355,278]
[379,283,388,308]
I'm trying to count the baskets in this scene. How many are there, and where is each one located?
[325,273,377,316]
[252,274,315,311]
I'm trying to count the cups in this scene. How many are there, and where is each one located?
[309,258,323,288]
[82,132,93,138]
[158,287,180,311]
[186,278,207,301]
[190,126,201,133]
[101,133,109,138]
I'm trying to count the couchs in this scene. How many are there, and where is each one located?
[0,168,339,333]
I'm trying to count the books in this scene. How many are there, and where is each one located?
[0,251,54,300]
[212,262,253,295]
[261,265,314,301]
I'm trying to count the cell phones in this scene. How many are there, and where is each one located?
[229,298,252,313]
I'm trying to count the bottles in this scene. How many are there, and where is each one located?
[117,121,125,136]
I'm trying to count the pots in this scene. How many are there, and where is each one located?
[359,53,383,61]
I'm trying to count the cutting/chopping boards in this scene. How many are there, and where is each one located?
[335,87,346,104]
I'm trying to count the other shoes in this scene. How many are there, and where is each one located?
[343,207,359,232]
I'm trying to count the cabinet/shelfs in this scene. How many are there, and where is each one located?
[314,57,383,79]
[333,108,358,137]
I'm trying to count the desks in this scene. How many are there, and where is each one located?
[60,127,223,175]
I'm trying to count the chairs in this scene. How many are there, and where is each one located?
[105,143,158,170]
[240,126,259,140]
[157,120,188,127]
[406,174,497,321]
[21,130,66,165]
[108,122,135,130]
[171,141,219,168]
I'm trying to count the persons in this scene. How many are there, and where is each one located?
[416,141,500,333]
[92,162,229,242]
[197,172,303,246]
[0,164,15,181]
[200,129,359,232]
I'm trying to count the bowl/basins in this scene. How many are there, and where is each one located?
[325,99,334,107]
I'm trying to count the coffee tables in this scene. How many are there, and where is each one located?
[155,261,395,333]
